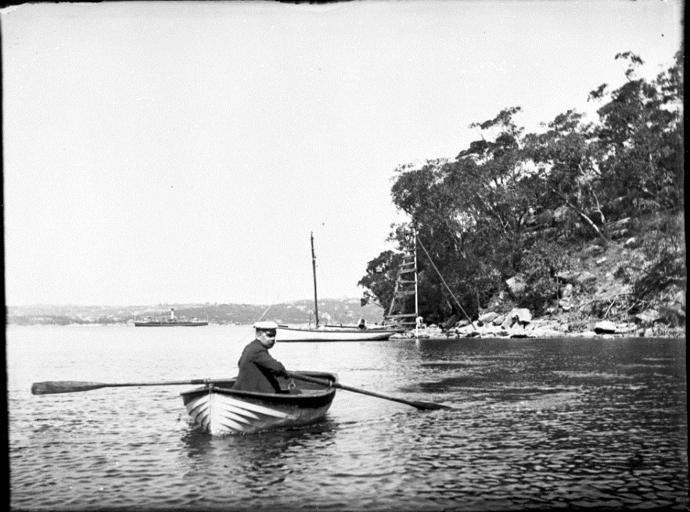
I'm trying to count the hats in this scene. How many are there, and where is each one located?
[253,321,279,335]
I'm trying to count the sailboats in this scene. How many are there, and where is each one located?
[257,231,405,342]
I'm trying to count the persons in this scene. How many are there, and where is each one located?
[231,320,302,395]
[358,319,368,330]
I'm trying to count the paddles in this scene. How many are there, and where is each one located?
[31,379,232,394]
[286,373,451,411]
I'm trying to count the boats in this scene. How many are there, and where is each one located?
[135,322,208,327]
[180,372,339,436]
[593,319,637,333]
